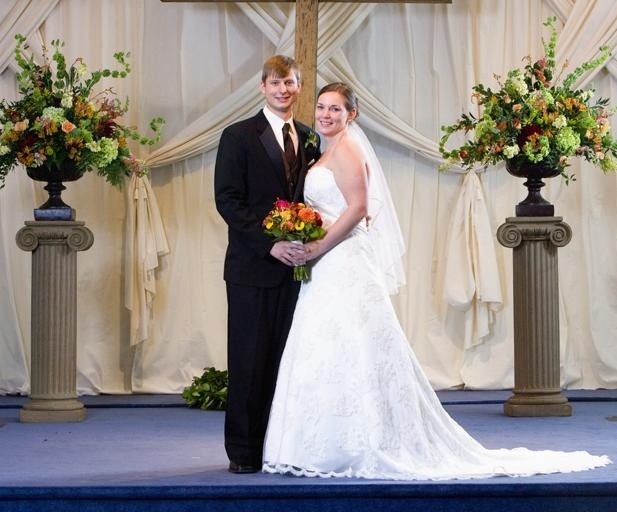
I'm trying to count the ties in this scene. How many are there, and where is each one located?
[282,122,299,205]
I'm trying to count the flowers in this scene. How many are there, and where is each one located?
[0,31,165,193]
[437,15,617,184]
[262,197,328,284]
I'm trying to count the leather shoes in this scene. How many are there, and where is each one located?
[230,459,258,474]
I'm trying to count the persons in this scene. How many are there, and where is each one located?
[214,56,322,474]
[261,82,615,479]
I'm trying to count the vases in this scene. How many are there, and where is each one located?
[26,163,88,222]
[505,151,563,220]
